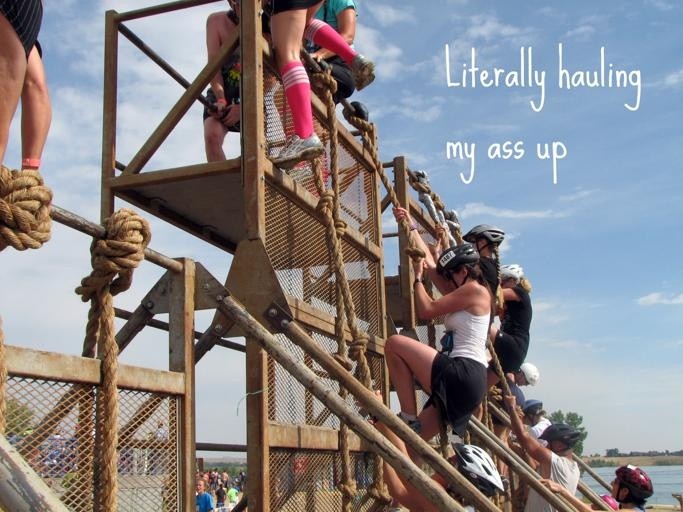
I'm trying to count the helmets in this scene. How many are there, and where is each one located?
[615,464,654,501]
[435,244,481,274]
[462,225,505,247]
[591,494,620,512]
[520,362,540,387]
[500,264,524,285]
[451,441,506,506]
[537,424,581,448]
[521,399,542,413]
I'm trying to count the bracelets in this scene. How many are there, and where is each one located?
[20,156,42,170]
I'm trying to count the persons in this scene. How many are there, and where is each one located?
[190,466,246,511]
[0,0,54,174]
[203,1,376,169]
[357,207,654,512]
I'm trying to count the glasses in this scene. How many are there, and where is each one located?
[443,265,462,282]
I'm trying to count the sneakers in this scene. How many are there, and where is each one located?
[271,131,325,170]
[372,412,423,437]
[350,53,375,91]
